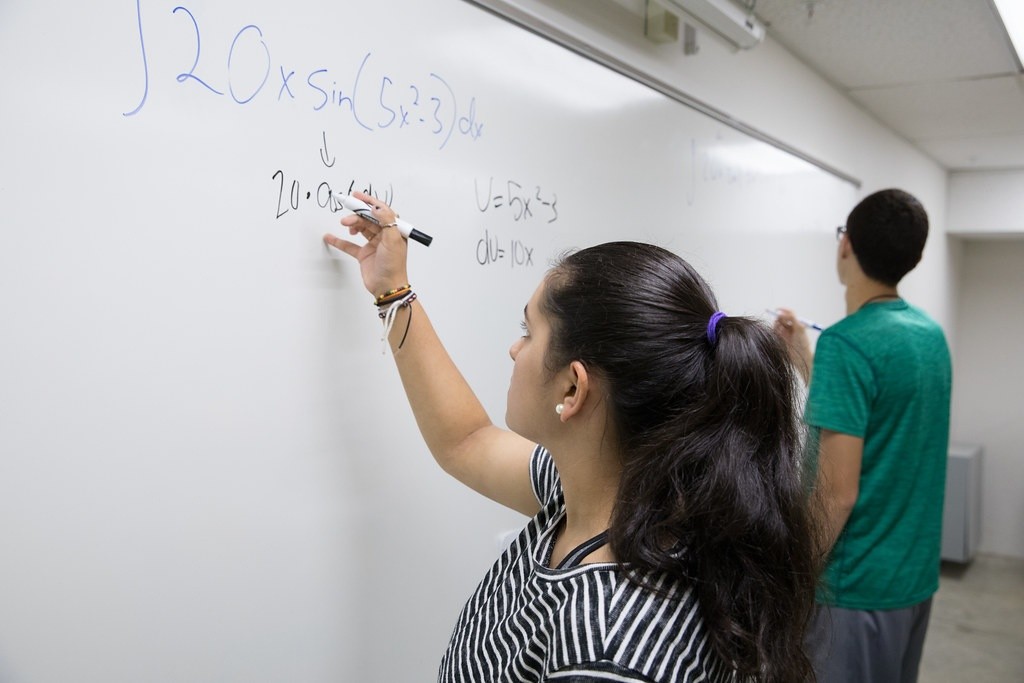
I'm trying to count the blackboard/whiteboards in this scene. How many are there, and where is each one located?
[0,0,876,683]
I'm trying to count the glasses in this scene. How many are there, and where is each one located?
[837,225,851,240]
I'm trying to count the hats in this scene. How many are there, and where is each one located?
[846,189,928,282]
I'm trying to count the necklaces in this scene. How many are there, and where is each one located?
[860,294,898,307]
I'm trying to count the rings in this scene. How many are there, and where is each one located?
[368,233,377,241]
[379,223,398,230]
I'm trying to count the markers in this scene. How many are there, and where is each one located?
[328,190,433,247]
[764,306,823,331]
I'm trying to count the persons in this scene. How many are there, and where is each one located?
[773,189,952,683]
[324,191,821,683]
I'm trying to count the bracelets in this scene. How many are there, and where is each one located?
[374,284,417,349]
